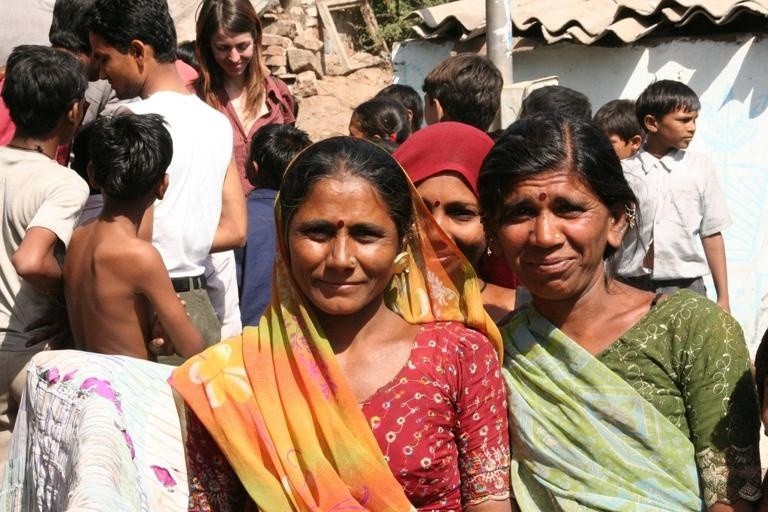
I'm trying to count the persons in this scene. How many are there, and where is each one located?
[476,112,763,511]
[348,97,411,152]
[605,81,731,315]
[753,331,768,508]
[516,84,593,120]
[187,1,298,203]
[0,0,248,429]
[423,52,504,127]
[169,136,515,512]
[594,98,648,161]
[377,82,424,137]
[235,122,316,326]
